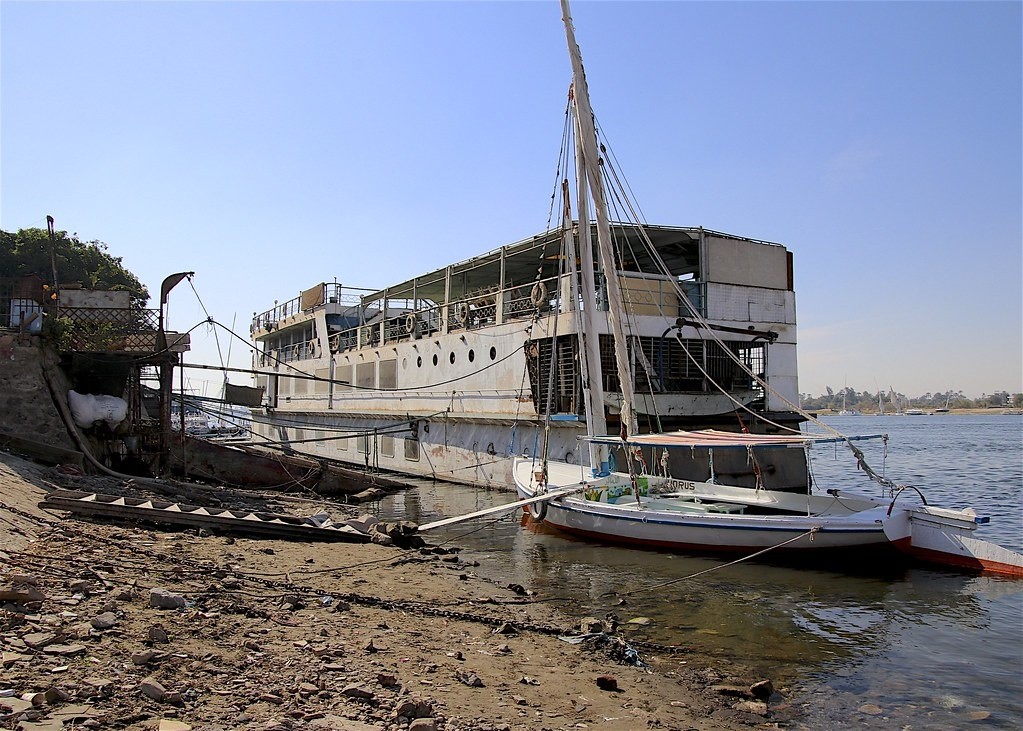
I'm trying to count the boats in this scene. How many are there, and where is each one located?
[250,220,888,494]
[905,409,924,415]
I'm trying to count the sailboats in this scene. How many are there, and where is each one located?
[534,1,1023,579]
[874,392,885,416]
[839,376,862,416]
[890,386,904,416]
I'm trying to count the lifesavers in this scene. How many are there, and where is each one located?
[308,341,314,354]
[366,326,374,343]
[406,315,415,333]
[260,355,263,364]
[531,282,547,307]
[335,335,341,350]
[294,345,300,357]
[458,303,470,323]
[530,492,547,522]
